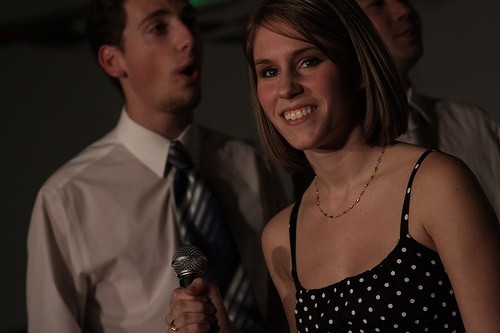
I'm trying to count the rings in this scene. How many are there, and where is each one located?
[168,319,177,331]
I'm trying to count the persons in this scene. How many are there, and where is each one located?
[356,0,500,224]
[164,0,500,333]
[25,0,291,333]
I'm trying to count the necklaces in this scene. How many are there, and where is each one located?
[313,148,385,220]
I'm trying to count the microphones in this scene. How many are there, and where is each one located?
[171,245,208,288]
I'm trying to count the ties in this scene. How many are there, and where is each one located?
[168,141,268,333]
